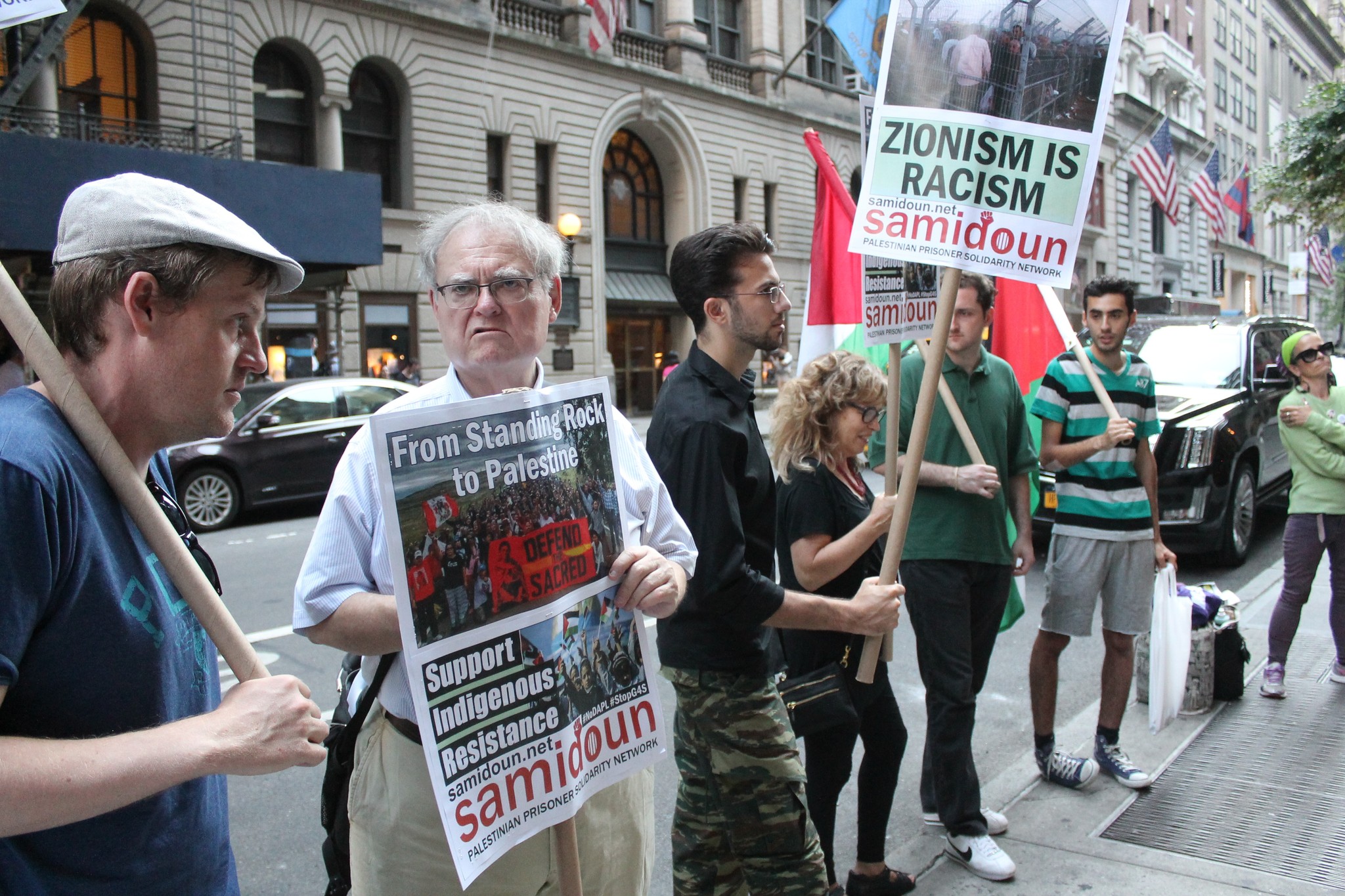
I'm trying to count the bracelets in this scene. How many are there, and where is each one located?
[953,463,958,493]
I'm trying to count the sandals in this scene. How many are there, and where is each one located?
[846,867,918,895]
[830,884,846,895]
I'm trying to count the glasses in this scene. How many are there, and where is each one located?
[844,398,885,425]
[431,275,543,308]
[717,281,785,303]
[1290,341,1336,365]
[151,477,224,600]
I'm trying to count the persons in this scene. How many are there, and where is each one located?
[288,197,703,896]
[662,349,680,383]
[769,342,793,391]
[0,169,329,896]
[557,619,647,730]
[0,345,28,393]
[1258,326,1345,699]
[886,13,1112,119]
[766,347,917,896]
[405,466,642,646]
[377,356,424,387]
[866,271,1046,881]
[643,224,908,895]
[1030,272,1178,791]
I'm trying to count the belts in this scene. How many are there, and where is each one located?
[378,706,424,745]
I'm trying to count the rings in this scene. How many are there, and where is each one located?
[1286,411,1292,422]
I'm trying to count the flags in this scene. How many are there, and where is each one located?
[795,130,916,378]
[523,595,625,688]
[1223,160,1258,246]
[990,277,1077,397]
[821,0,890,91]
[1303,220,1336,289]
[1191,144,1231,239]
[1128,114,1181,227]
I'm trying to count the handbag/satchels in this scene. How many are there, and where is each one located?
[1147,564,1193,734]
[774,663,859,738]
[322,646,361,896]
[1136,625,1216,715]
[1214,622,1251,699]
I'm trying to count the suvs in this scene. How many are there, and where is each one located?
[1027,311,1340,567]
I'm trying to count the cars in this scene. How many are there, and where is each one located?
[166,376,448,533]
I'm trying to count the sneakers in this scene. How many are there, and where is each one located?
[1259,662,1289,698]
[1092,734,1153,788]
[923,806,1008,835]
[1037,750,1101,788]
[945,830,1016,881]
[1331,659,1345,684]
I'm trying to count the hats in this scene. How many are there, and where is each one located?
[51,170,306,300]
[1282,330,1313,368]
